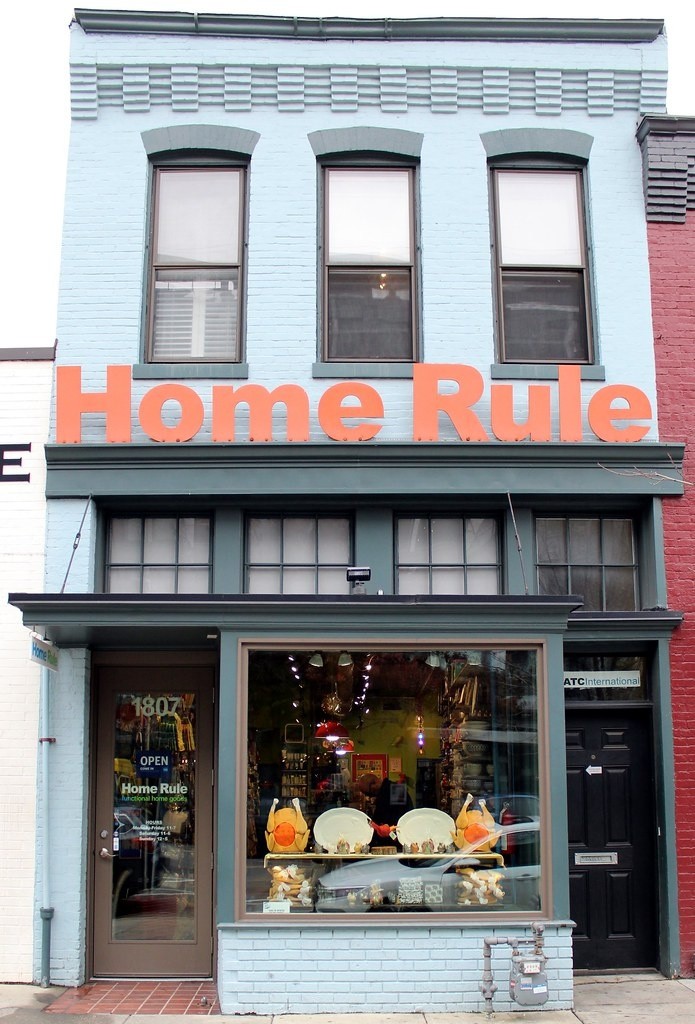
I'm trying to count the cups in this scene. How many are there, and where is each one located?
[302,789,306,796]
[302,777,306,784]
[291,777,294,784]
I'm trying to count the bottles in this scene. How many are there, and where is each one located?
[290,786,294,796]
[337,798,341,808]
[341,791,349,807]
[282,785,286,796]
[294,786,298,796]
[282,773,286,783]
[299,775,302,784]
[299,788,302,796]
[286,787,290,796]
[285,761,303,770]
[286,775,290,784]
[294,774,298,784]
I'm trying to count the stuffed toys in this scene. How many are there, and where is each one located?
[450,793,499,852]
[455,867,505,906]
[267,864,312,899]
[265,797,310,853]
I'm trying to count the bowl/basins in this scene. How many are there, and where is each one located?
[484,782,494,792]
[464,764,482,776]
[471,797,485,809]
[461,780,481,792]
[462,742,486,756]
[489,797,494,808]
[487,765,494,776]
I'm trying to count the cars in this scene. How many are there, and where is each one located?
[476,795,541,859]
[312,816,541,913]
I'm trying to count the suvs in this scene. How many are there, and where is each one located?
[111,806,160,909]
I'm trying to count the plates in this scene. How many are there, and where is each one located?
[314,808,374,852]
[396,808,456,852]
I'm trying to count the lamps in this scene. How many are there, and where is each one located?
[326,742,355,755]
[425,654,440,667]
[346,567,371,588]
[467,654,481,665]
[314,720,350,741]
[291,663,301,672]
[295,673,302,679]
[309,653,323,667]
[362,707,369,713]
[338,654,353,666]
[292,700,299,708]
[288,654,296,662]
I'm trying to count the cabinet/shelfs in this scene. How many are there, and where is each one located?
[438,664,504,801]
[263,853,508,912]
[279,759,308,815]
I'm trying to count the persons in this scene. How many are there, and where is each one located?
[356,773,415,854]
